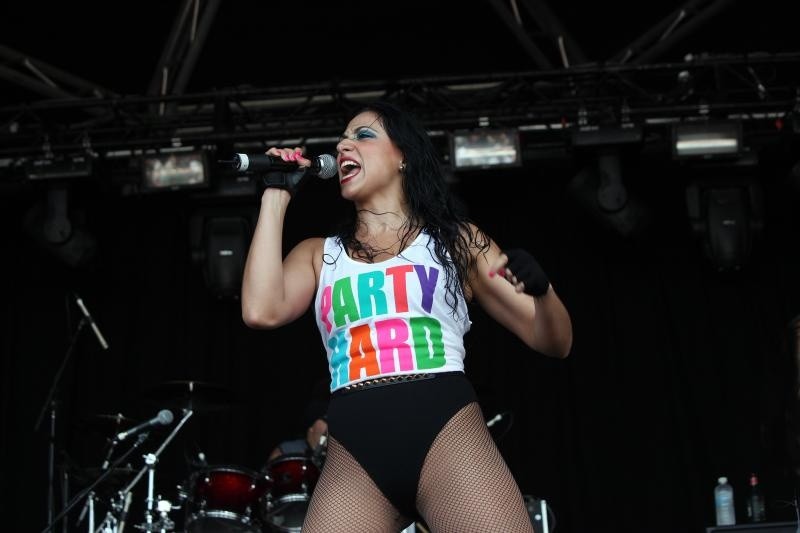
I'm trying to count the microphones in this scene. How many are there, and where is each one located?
[218,154,338,179]
[113,409,174,441]
[76,298,109,349]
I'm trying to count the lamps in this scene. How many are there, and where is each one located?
[448,129,522,171]
[140,154,207,189]
[672,126,742,158]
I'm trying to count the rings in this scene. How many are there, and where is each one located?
[309,428,313,432]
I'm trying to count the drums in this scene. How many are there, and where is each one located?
[263,457,316,533]
[184,469,256,533]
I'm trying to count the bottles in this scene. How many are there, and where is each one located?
[714,477,735,525]
[745,472,767,524]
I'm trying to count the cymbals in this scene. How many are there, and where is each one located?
[158,380,216,386]
[101,466,142,475]
[91,412,144,422]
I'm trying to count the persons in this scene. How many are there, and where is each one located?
[305,381,329,450]
[241,101,573,533]
